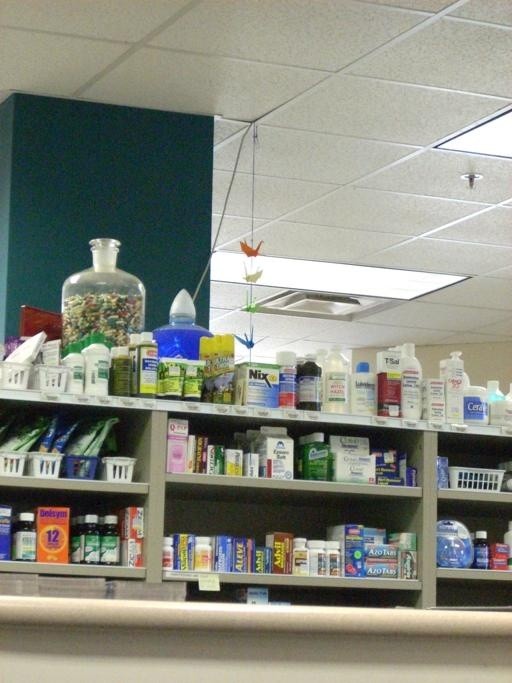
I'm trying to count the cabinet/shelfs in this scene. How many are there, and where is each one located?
[2,363,512,605]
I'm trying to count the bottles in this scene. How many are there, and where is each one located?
[193,536,211,572]
[70,515,121,565]
[468,531,488,567]
[291,537,340,579]
[63,330,158,397]
[276,341,512,432]
[502,519,512,571]
[9,512,38,562]
[297,432,332,482]
[162,536,174,570]
